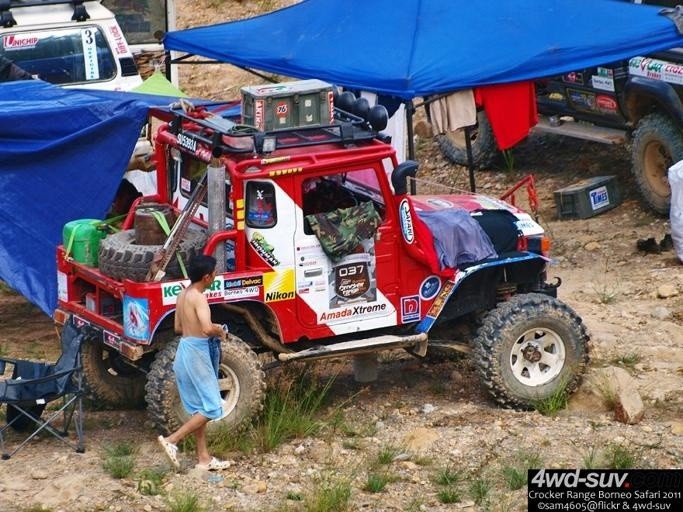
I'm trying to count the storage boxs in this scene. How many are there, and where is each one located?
[552,173,624,222]
[237,77,334,139]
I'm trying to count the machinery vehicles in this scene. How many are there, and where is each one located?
[53,77,591,450]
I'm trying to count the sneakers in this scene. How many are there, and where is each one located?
[638,238,660,253]
[660,234,672,250]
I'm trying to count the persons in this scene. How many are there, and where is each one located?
[666,157,683,264]
[159,255,231,470]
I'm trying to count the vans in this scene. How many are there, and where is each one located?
[0,1,152,168]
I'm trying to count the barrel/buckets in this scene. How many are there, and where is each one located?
[63,219,106,267]
[135,206,172,245]
[349,353,378,383]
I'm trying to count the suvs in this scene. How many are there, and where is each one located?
[423,0,683,220]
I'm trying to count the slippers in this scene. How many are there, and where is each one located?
[157,435,182,472]
[195,456,231,471]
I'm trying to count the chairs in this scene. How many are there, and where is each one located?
[0,312,101,463]
